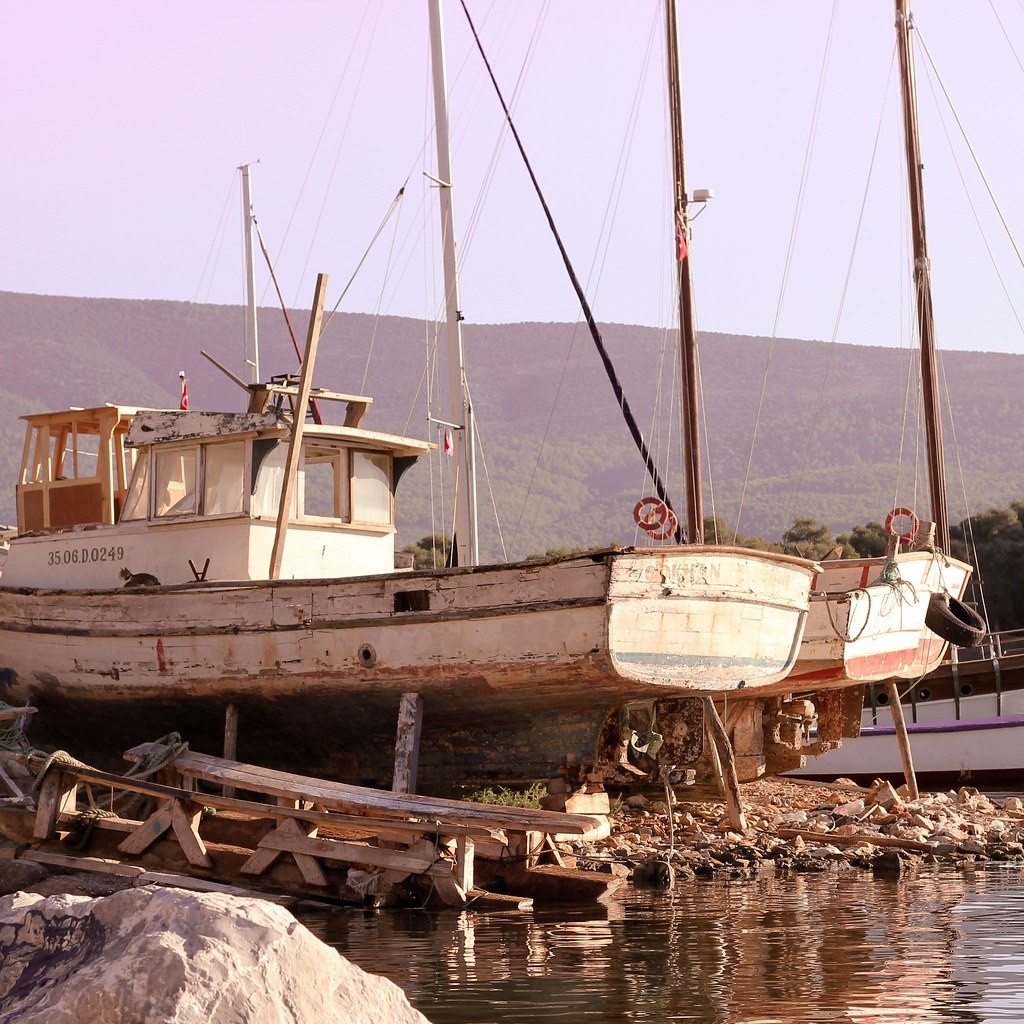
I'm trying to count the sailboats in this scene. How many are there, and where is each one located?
[1,0,1024,783]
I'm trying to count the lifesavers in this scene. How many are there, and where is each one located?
[633,497,669,531]
[885,507,919,544]
[925,593,986,649]
[643,508,678,540]
